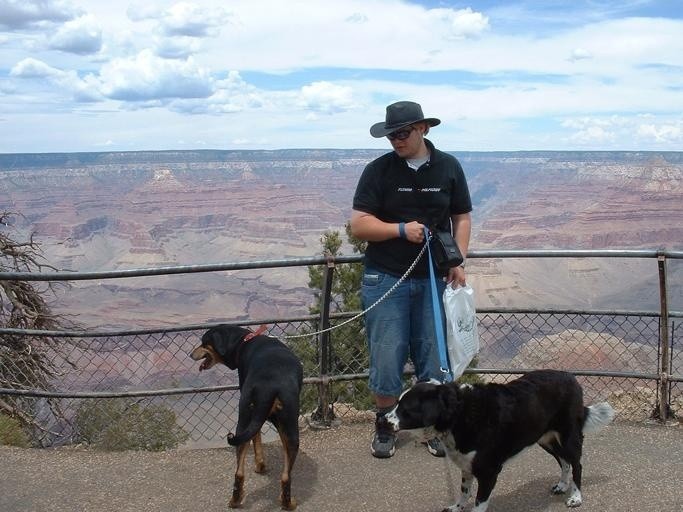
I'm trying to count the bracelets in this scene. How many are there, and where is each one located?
[399,220,406,241]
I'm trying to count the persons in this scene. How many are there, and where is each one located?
[349,100,473,459]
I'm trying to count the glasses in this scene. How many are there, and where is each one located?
[385,125,417,140]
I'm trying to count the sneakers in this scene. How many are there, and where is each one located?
[427,436,447,458]
[370,412,398,458]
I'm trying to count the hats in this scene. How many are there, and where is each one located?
[370,101,440,138]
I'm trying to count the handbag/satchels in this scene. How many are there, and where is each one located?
[428,231,464,270]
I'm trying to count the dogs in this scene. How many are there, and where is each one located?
[188,322,304,511]
[378,368,615,512]
